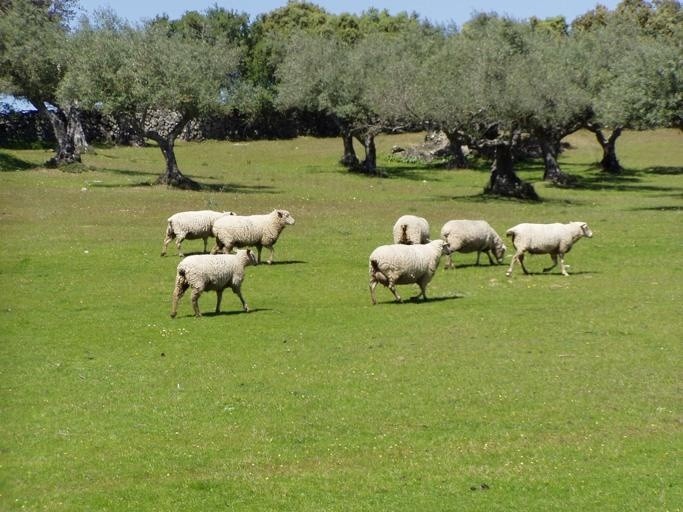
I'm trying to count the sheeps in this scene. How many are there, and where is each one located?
[392,215,430,245]
[160,210,237,258]
[440,219,507,270]
[209,207,296,265]
[506,220,593,277]
[170,246,258,318]
[368,238,452,305]
